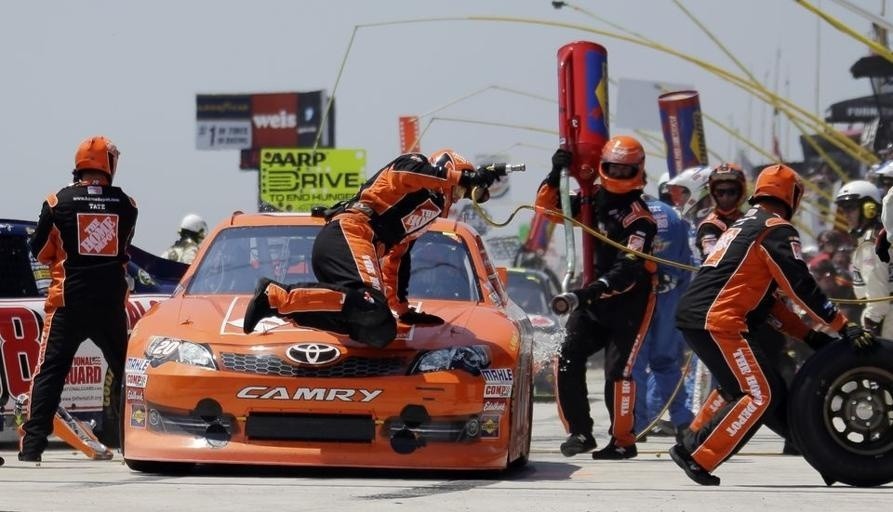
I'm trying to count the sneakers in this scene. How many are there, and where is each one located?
[669,440,720,486]
[244,277,274,335]
[560,432,597,457]
[592,435,637,459]
[18,450,42,461]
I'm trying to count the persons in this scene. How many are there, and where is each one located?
[242,149,500,349]
[161,214,208,266]
[636,160,892,487]
[18,136,137,462]
[534,135,659,461]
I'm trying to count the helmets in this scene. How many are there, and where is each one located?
[73,135,120,183]
[429,149,473,204]
[834,181,882,238]
[657,164,806,219]
[817,229,855,271]
[181,213,208,245]
[598,135,647,195]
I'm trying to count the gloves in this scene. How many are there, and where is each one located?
[804,330,838,351]
[546,149,572,188]
[458,166,500,189]
[399,308,444,324]
[875,230,891,263]
[839,321,875,351]
[567,281,608,311]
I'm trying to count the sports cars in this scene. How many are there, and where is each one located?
[495,264,568,397]
[119,199,534,471]
[1,215,187,419]
